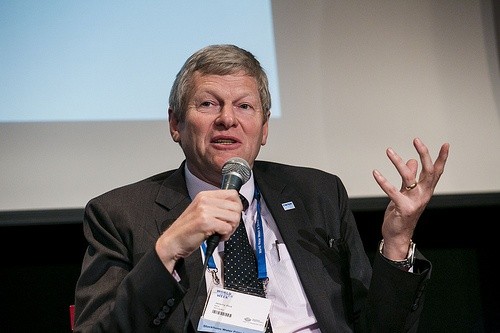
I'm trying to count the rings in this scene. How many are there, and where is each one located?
[404,182,417,191]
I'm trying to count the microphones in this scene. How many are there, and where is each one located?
[206,157,252,257]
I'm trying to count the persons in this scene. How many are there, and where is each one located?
[72,45,450,333]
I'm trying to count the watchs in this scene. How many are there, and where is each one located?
[379,239,416,269]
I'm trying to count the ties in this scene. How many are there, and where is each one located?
[223,211,265,299]
